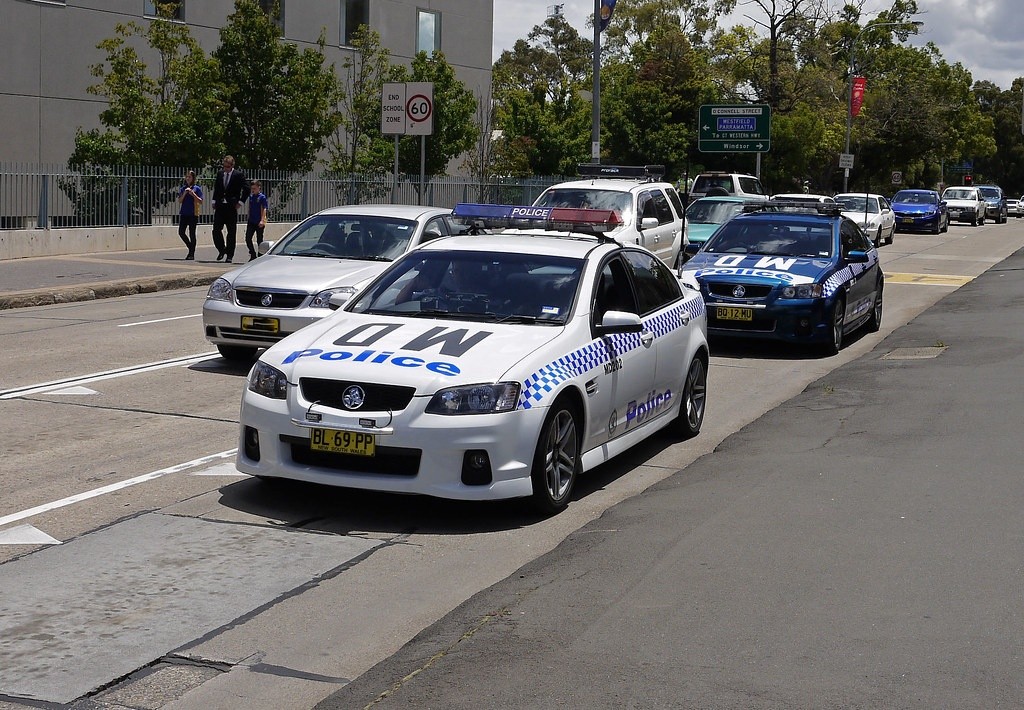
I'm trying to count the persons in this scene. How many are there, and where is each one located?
[245,180,268,262]
[395,260,481,314]
[212,155,251,262]
[676,172,693,208]
[803,181,811,194]
[178,171,203,260]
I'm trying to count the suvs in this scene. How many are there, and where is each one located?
[515,162,689,273]
[688,171,779,213]
[1020,195,1024,214]
[971,186,1009,223]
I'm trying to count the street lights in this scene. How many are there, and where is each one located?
[844,21,924,190]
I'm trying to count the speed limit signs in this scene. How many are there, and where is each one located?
[404,81,435,136]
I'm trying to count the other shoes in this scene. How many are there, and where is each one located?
[248,253,257,262]
[226,257,232,263]
[186,254,194,260]
[217,248,226,260]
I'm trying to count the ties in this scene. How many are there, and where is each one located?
[225,173,229,190]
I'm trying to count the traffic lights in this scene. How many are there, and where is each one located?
[963,176,972,186]
[697,104,771,153]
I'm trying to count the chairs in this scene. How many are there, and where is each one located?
[316,223,433,257]
[439,260,621,318]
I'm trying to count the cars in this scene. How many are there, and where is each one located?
[202,204,467,362]
[680,196,783,264]
[827,192,898,245]
[770,193,834,216]
[888,189,951,234]
[938,185,986,227]
[1006,198,1023,218]
[674,201,882,360]
[238,204,708,519]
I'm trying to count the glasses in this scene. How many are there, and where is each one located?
[223,165,232,168]
